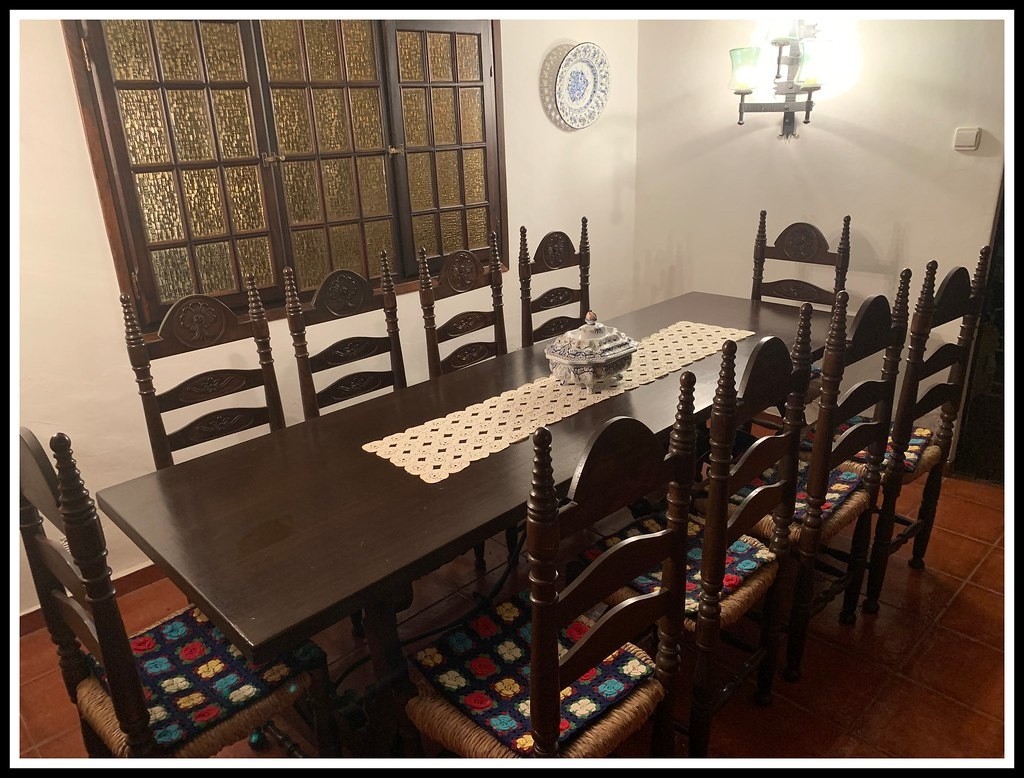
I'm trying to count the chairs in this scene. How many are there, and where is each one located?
[19,209,993,759]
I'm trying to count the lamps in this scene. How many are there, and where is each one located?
[728,20,832,137]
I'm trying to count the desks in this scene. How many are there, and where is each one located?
[98,290,859,759]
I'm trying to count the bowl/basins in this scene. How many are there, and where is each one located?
[544,308,644,394]
